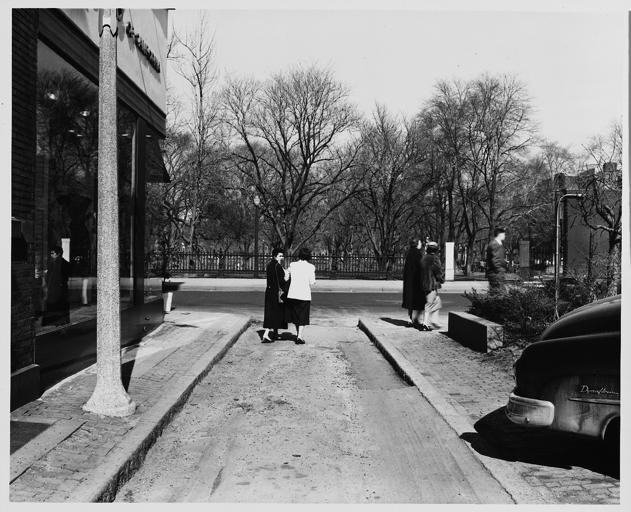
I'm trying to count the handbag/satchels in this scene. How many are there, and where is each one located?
[278,287,284,303]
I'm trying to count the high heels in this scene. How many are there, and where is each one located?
[262,336,274,343]
[408,319,442,331]
[296,336,306,344]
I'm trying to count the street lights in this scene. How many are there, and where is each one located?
[253,192,262,279]
[552,192,586,321]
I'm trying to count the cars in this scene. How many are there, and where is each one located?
[504,295,620,450]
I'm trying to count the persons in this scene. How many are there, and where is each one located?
[420,241,443,332]
[283,248,316,344]
[485,228,508,297]
[401,239,424,327]
[262,247,290,342]
[41,245,71,327]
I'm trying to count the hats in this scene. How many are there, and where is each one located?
[425,242,440,247]
